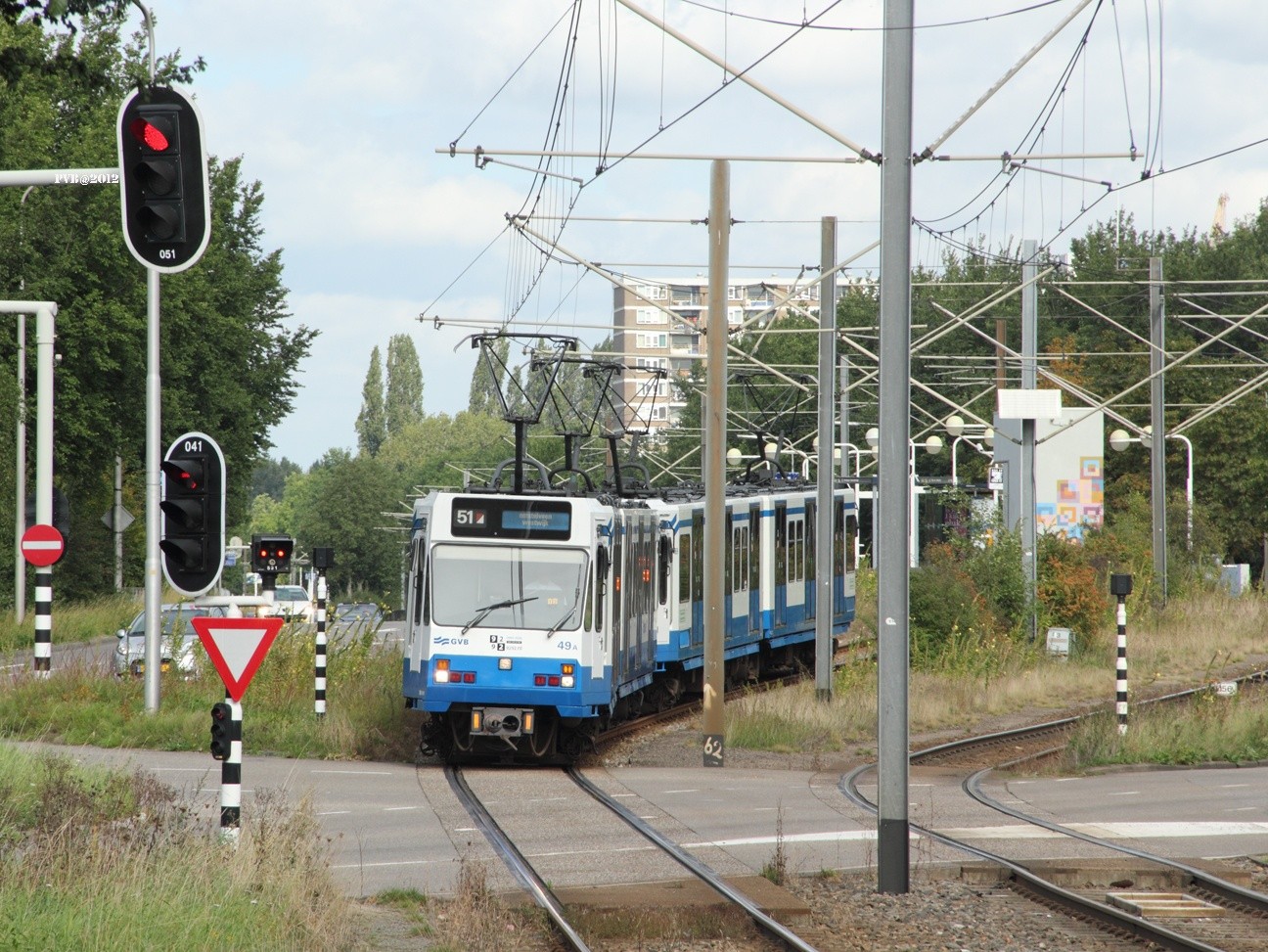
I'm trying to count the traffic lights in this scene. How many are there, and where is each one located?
[117,84,211,274]
[158,431,227,598]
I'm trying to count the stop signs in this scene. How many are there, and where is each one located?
[20,523,65,568]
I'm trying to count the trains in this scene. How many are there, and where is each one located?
[395,328,867,762]
